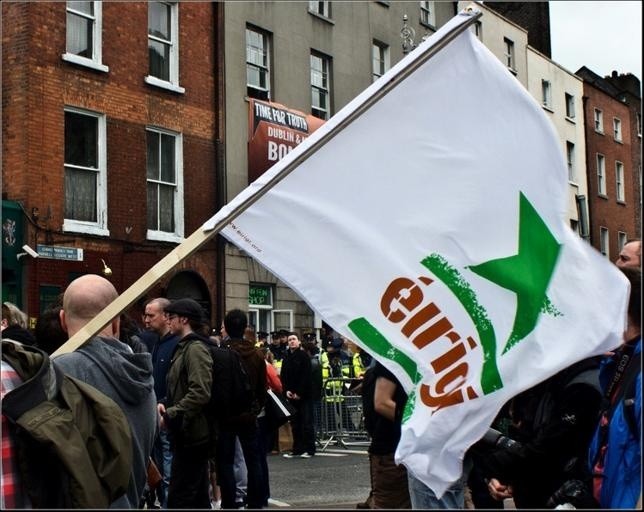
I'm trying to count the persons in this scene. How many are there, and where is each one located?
[2,274,283,509]
[362,359,412,508]
[589,267,641,509]
[406,356,605,508]
[615,239,640,271]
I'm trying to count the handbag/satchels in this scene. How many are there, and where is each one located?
[258,388,291,435]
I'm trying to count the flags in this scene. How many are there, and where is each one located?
[200,27,632,501]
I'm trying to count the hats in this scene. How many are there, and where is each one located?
[303,332,316,341]
[257,329,292,339]
[325,338,343,348]
[163,299,203,321]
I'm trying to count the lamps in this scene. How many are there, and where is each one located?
[99,255,116,277]
